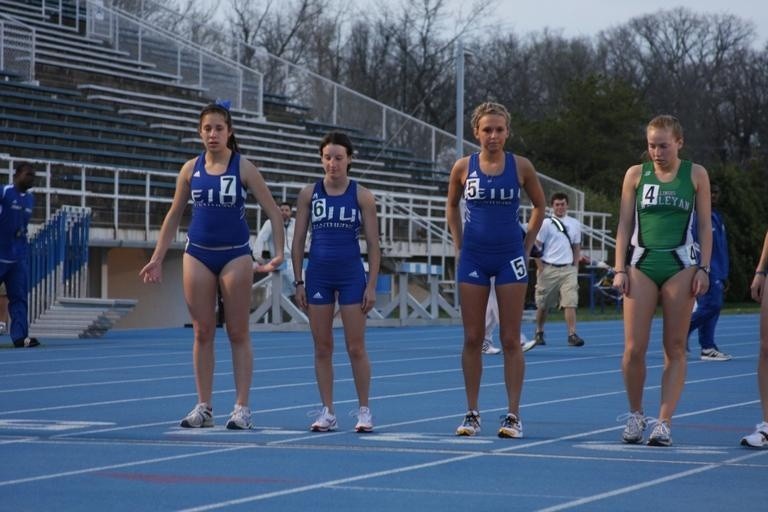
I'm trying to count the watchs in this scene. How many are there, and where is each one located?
[698,265,712,273]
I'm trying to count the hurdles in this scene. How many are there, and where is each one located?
[26,205,137,338]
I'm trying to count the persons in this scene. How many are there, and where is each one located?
[482,275,536,355]
[536,193,584,345]
[740,231,768,447]
[613,115,713,445]
[140,98,285,429]
[447,103,545,438]
[291,133,379,432]
[0,164,41,347]
[685,181,732,361]
[252,202,305,288]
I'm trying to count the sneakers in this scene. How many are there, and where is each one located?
[740,422,768,448]
[535,331,545,345]
[498,413,522,438]
[355,407,373,432]
[309,406,338,431]
[700,349,730,361]
[616,411,647,443]
[643,417,673,446]
[568,335,584,346]
[15,338,40,347]
[455,411,481,435]
[226,406,254,430]
[179,403,214,427]
[522,341,537,352]
[481,342,502,354]
